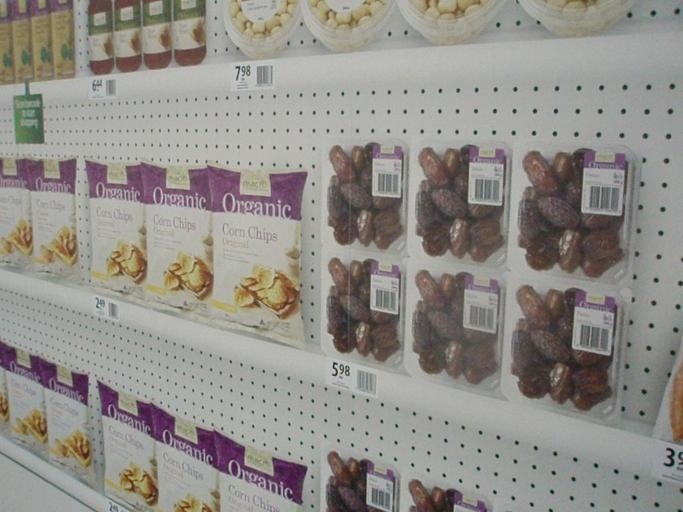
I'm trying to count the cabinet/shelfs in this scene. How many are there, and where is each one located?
[0,2,683,512]
